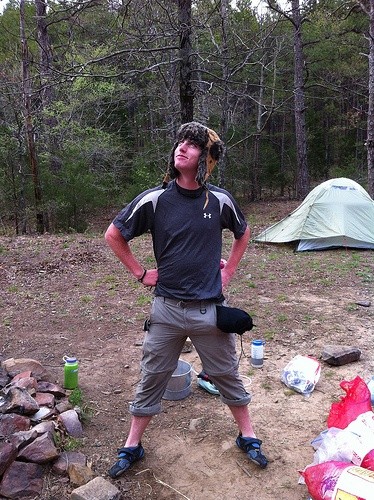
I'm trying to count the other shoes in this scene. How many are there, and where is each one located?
[109,440,146,478]
[236,432,268,468]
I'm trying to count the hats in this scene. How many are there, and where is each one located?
[161,121,225,190]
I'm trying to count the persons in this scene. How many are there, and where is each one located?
[104,120,270,479]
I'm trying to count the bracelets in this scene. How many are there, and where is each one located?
[138,268,147,283]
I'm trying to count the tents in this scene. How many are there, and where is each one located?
[251,176,374,253]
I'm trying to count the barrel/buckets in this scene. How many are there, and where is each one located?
[162,360,193,400]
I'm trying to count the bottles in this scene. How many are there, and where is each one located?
[62,355,79,389]
[251,339,264,368]
